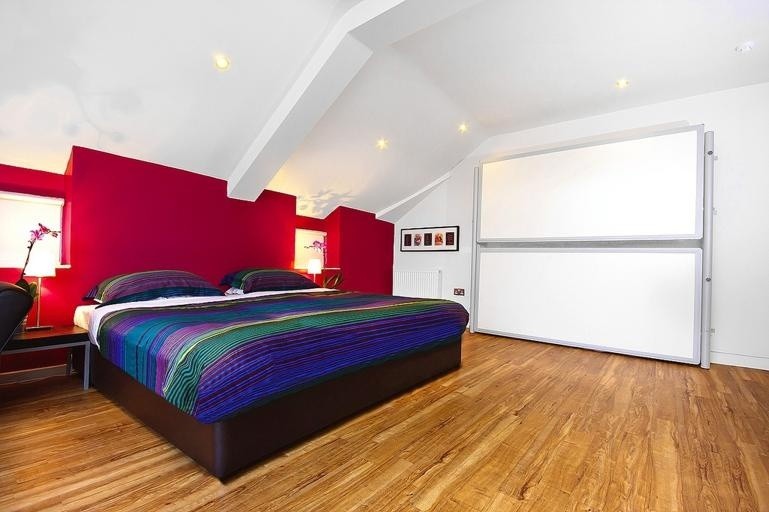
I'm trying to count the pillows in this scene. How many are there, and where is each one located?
[83,265,322,308]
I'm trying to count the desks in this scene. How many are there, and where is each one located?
[2,323,90,392]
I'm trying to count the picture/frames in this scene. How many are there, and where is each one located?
[399,225,461,252]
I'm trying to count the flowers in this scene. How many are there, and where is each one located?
[14,222,60,302]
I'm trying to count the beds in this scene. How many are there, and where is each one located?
[71,289,469,477]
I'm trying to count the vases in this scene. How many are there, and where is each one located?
[12,313,30,334]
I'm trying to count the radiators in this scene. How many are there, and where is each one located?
[392,270,443,300]
